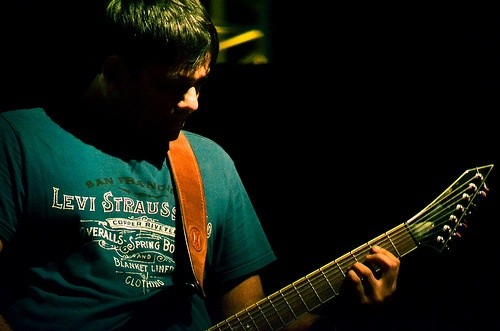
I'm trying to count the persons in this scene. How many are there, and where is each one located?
[1,0,401,331]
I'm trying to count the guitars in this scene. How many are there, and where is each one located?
[202,164,495,330]
